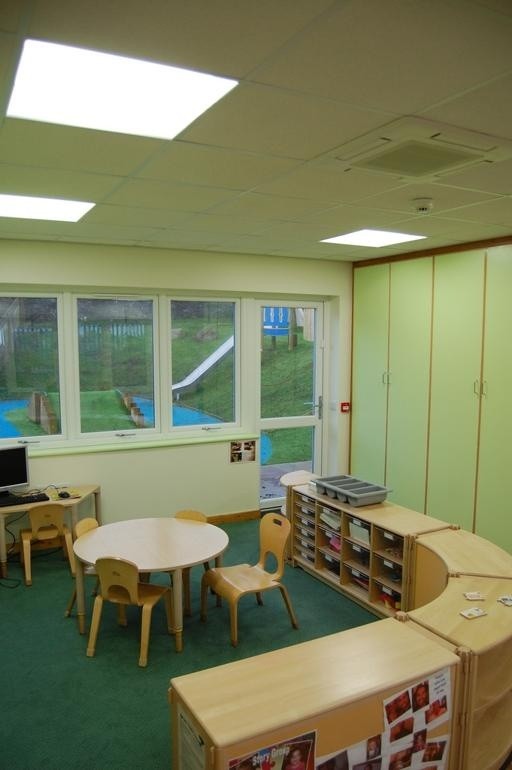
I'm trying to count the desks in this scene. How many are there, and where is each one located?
[396,571,512,764]
[281,468,315,571]
[411,528,509,619]
[289,478,454,621]
[167,616,459,764]
[2,484,102,574]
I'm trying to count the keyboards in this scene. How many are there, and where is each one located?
[1,492,50,507]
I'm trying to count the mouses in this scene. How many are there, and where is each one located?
[58,490,70,499]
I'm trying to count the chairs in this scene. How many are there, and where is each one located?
[19,499,84,584]
[83,559,171,669]
[172,509,228,616]
[202,511,292,645]
[66,516,110,632]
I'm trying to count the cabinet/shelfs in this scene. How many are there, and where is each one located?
[350,247,430,522]
[423,243,510,550]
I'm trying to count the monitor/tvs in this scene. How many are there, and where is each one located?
[0,444,30,491]
